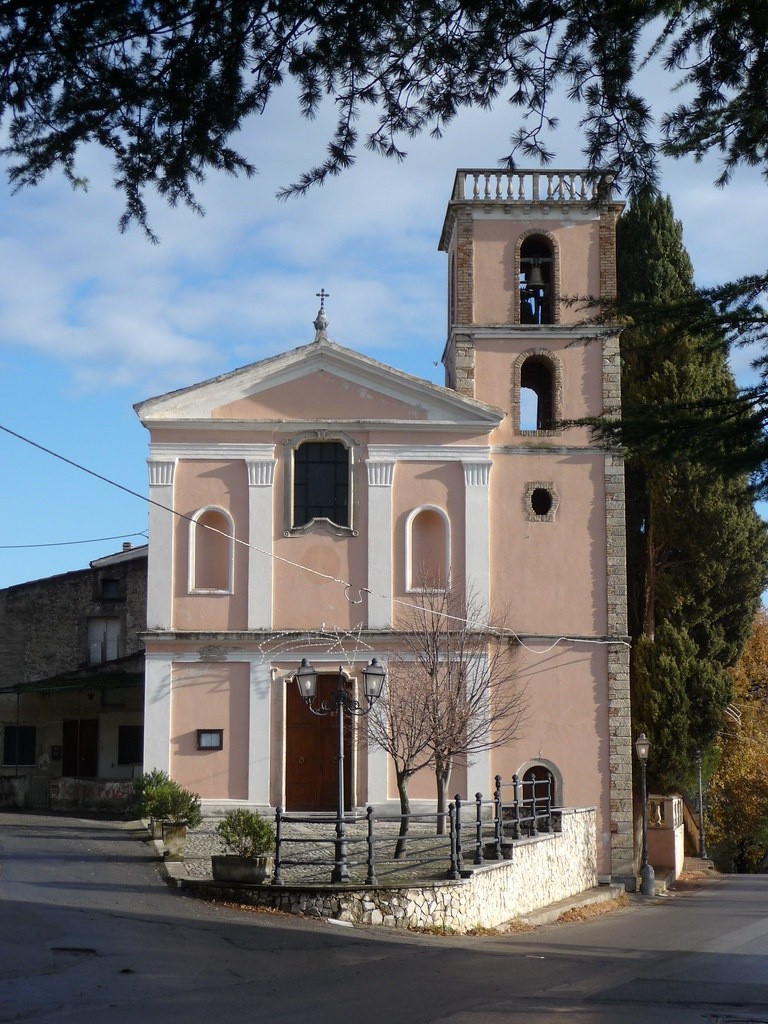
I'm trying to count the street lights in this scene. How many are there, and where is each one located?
[293,657,389,885]
[634,732,651,875]
[695,749,709,861]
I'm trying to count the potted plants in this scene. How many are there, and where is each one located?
[144,783,203,860]
[133,765,181,839]
[211,807,275,885]
